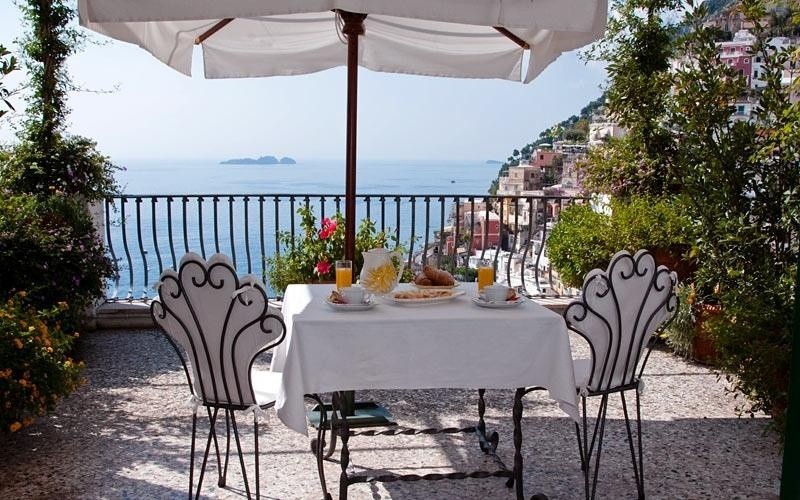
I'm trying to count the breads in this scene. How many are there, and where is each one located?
[414,265,456,287]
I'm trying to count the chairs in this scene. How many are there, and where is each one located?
[149,251,333,500]
[506,249,681,499]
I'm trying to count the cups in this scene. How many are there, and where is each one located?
[477,261,494,292]
[478,282,508,303]
[335,260,353,290]
[340,286,372,304]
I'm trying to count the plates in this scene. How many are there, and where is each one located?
[387,288,468,307]
[472,297,526,308]
[408,281,461,290]
[325,297,383,311]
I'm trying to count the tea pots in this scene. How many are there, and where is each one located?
[357,248,405,297]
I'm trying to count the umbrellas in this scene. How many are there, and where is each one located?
[77,0,608,416]
